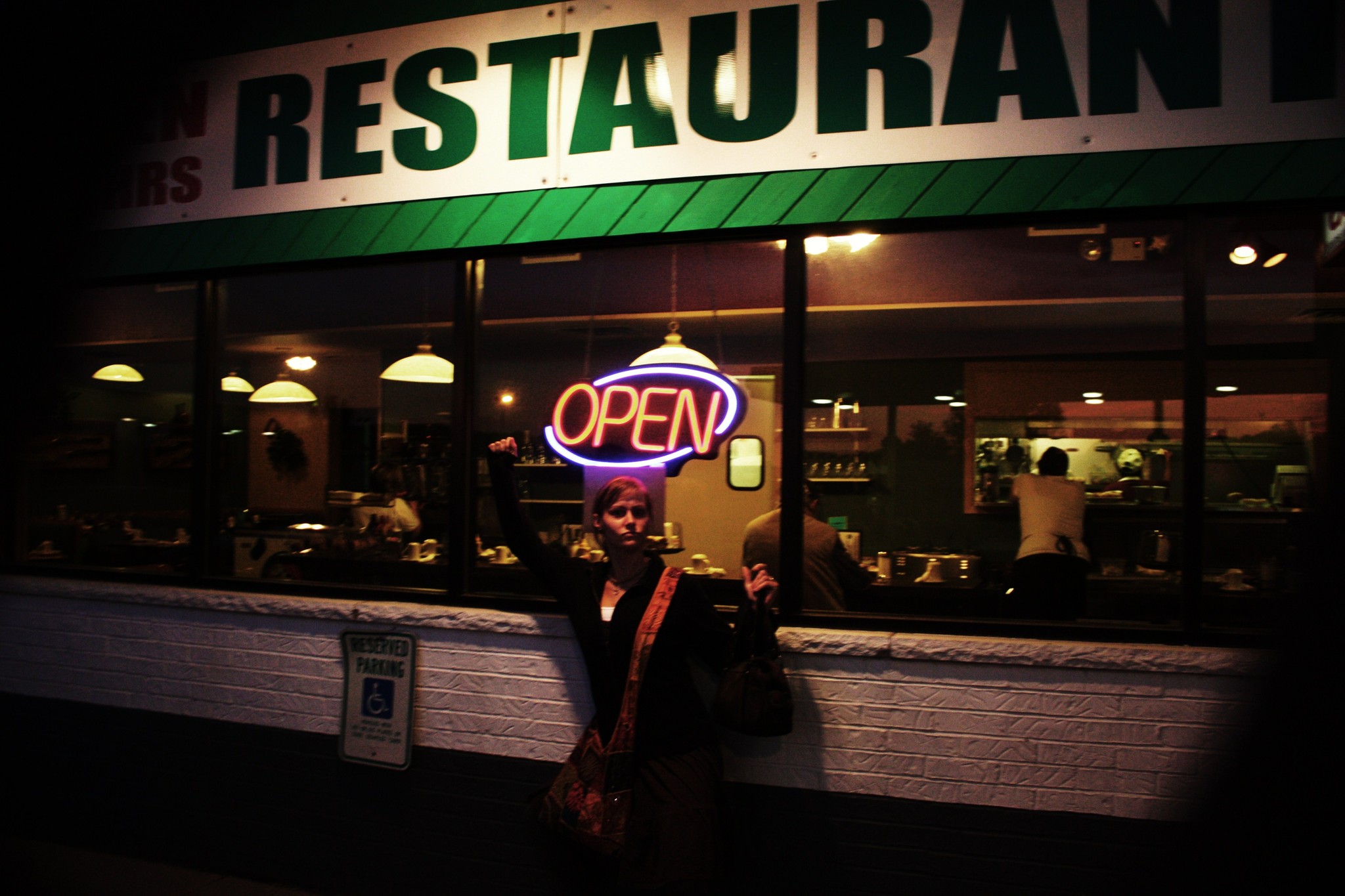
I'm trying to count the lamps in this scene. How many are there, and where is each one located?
[378,323,454,383]
[1228,234,1287,269]
[1080,237,1168,262]
[222,371,255,392]
[629,246,719,371]
[92,364,144,381]
[248,353,317,403]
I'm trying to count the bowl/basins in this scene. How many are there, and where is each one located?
[1101,560,1126,577]
[1134,486,1168,503]
[590,550,604,561]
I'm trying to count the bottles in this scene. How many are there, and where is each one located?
[1150,530,1167,562]
[475,537,482,553]
[671,536,680,548]
[569,540,580,557]
[580,539,590,557]
[808,463,865,477]
[804,400,866,428]
[521,443,561,465]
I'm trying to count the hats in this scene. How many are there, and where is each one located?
[1116,449,1146,473]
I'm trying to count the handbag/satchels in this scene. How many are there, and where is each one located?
[709,586,796,735]
[535,724,634,854]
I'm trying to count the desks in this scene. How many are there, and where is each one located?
[1085,557,1297,620]
[283,548,526,588]
[860,556,983,614]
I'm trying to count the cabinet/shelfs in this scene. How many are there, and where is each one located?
[513,463,585,503]
[803,427,870,481]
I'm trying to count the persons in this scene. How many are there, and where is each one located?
[350,468,422,538]
[484,437,794,896]
[1011,447,1092,641]
[1103,448,1151,497]
[742,480,877,630]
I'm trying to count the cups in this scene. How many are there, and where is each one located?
[692,554,710,572]
[409,539,438,559]
[495,546,506,562]
[926,563,942,581]
[878,558,892,578]
[1227,569,1242,588]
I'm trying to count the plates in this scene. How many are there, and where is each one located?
[1086,497,1124,502]
[1220,585,1254,593]
[490,561,515,566]
[400,554,441,562]
[648,548,685,554]
[686,571,714,577]
[923,580,948,584]
